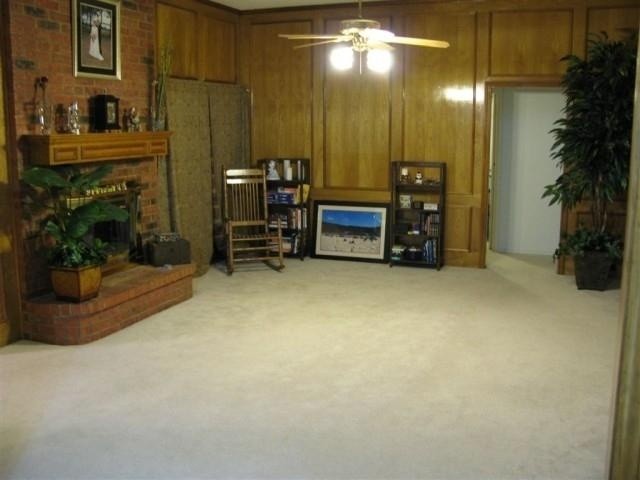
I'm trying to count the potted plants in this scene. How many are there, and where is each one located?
[539,29,640,291]
[19,162,129,303]
[150,36,173,132]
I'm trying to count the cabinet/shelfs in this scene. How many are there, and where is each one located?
[389,161,446,272]
[258,158,313,261]
[20,130,175,168]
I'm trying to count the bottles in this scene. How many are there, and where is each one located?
[31,75,54,136]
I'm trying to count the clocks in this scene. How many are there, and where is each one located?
[89,94,122,133]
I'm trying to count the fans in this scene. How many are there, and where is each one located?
[278,0,451,52]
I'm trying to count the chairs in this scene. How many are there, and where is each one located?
[221,162,286,276]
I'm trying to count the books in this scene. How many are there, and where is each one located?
[268,207,307,254]
[297,160,307,182]
[266,187,302,205]
[420,213,439,262]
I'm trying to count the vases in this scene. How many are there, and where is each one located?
[34,76,52,135]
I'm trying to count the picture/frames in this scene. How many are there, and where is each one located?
[311,200,391,264]
[73,0,121,81]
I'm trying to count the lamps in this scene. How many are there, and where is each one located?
[330,28,396,73]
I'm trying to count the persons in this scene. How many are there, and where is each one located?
[88,11,104,61]
